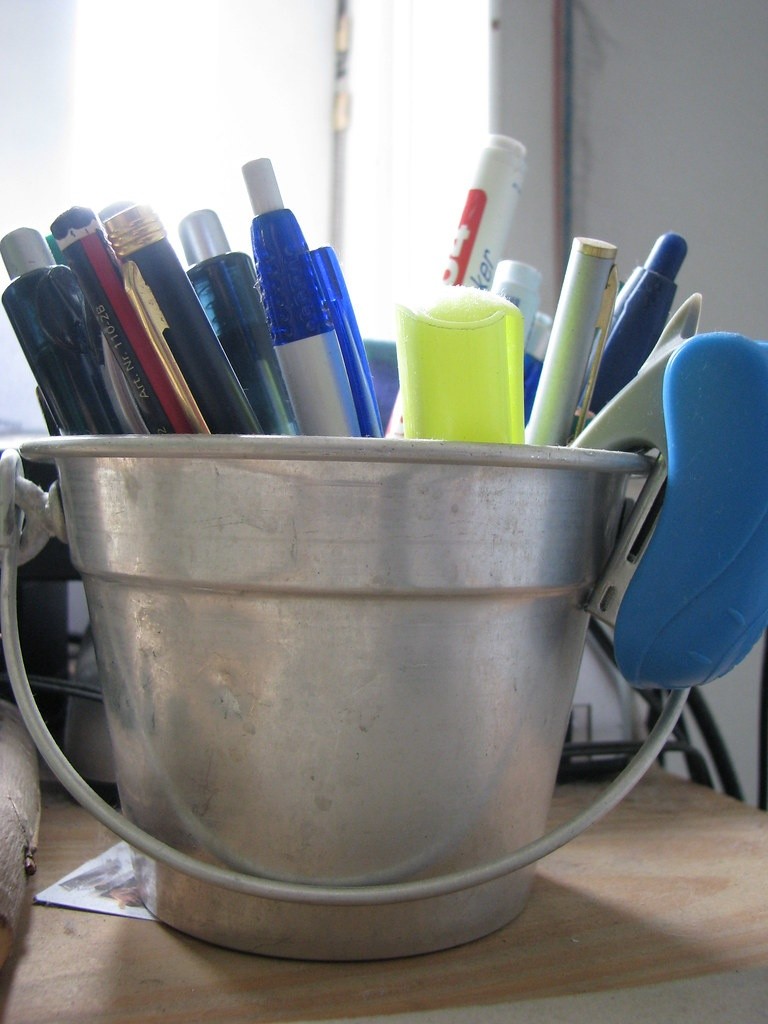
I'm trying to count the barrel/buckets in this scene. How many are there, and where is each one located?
[1,433,694,962]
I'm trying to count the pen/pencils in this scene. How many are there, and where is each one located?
[0,133,691,452]
